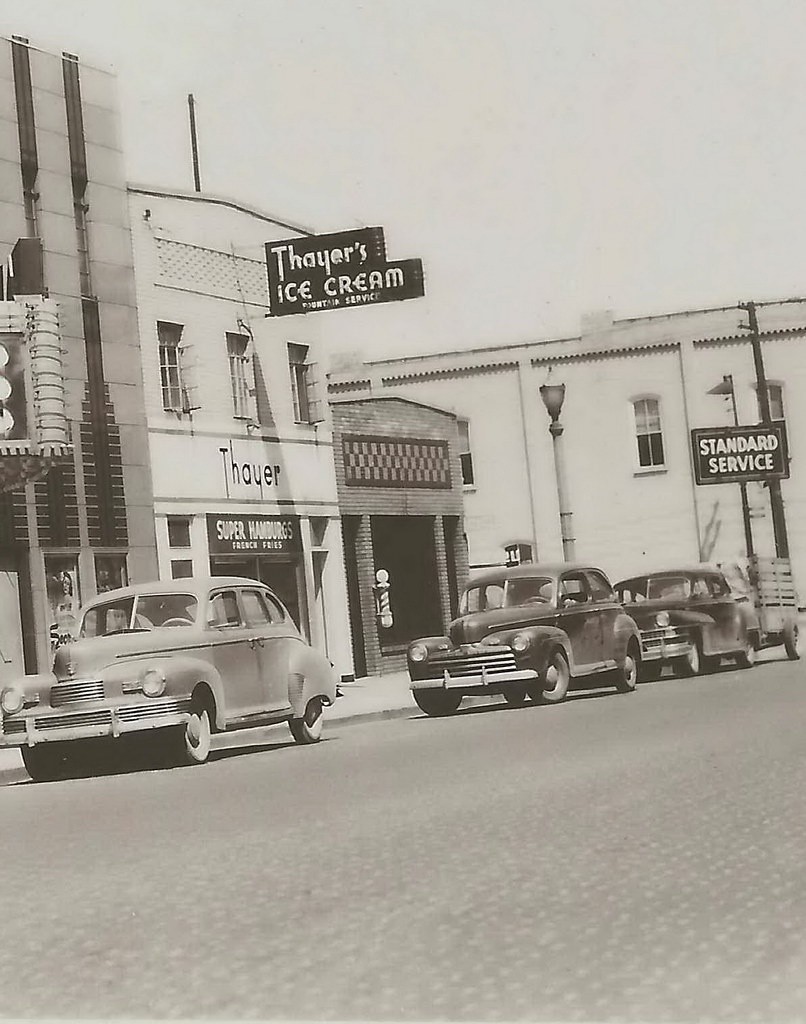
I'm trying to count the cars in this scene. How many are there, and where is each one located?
[0,574,347,780]
[610,568,763,684]
[405,561,650,717]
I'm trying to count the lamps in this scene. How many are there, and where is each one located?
[372,569,394,630]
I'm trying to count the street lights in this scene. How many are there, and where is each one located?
[537,364,582,593]
[705,375,756,556]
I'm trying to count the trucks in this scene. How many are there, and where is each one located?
[690,556,804,661]
